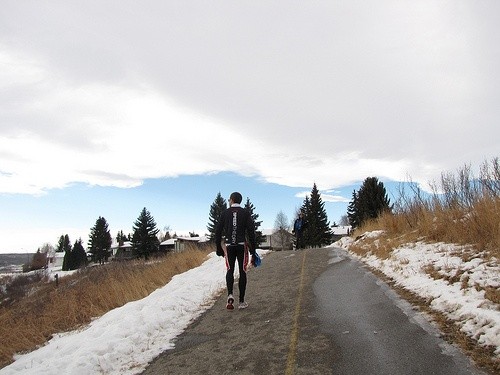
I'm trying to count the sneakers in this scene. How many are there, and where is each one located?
[238,302,248,308]
[226,294,234,311]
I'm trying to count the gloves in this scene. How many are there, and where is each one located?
[217,248,224,257]
[249,248,255,255]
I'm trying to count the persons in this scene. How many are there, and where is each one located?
[216,192,256,309]
[294,212,306,251]
